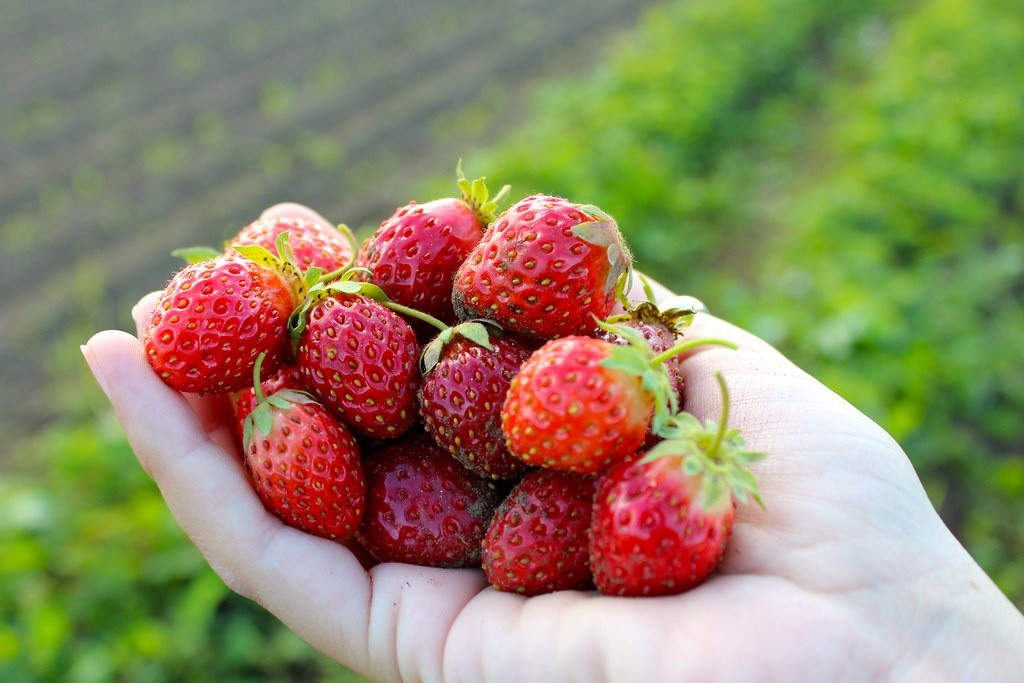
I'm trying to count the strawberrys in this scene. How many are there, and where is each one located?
[144,195,737,595]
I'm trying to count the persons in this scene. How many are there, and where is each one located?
[77,272,1024,683]
